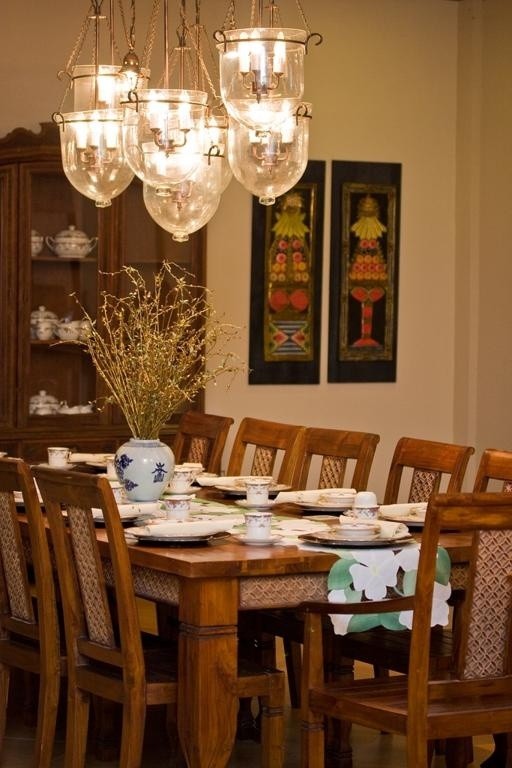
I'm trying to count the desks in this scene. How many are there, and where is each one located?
[0,458,473,764]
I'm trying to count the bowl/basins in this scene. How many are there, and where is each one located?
[335,525,376,538]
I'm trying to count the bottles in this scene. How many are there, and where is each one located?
[31,306,60,339]
[29,389,60,415]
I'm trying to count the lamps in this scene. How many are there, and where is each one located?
[50,1,325,242]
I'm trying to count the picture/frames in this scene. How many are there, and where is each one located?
[327,160,404,384]
[248,157,327,384]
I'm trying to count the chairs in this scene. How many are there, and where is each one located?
[285,428,381,497]
[29,463,291,766]
[285,438,474,735]
[2,454,88,768]
[225,418,304,490]
[169,412,232,479]
[473,449,512,493]
[309,491,511,768]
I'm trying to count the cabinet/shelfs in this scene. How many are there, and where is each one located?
[1,118,208,432]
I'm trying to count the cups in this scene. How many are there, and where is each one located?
[35,318,94,341]
[100,455,203,518]
[33,402,94,417]
[244,512,273,538]
[46,447,71,464]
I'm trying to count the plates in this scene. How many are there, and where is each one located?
[31,229,44,255]
[297,532,416,548]
[233,533,287,547]
[32,454,108,476]
[7,490,128,525]
[204,473,428,527]
[124,524,230,543]
